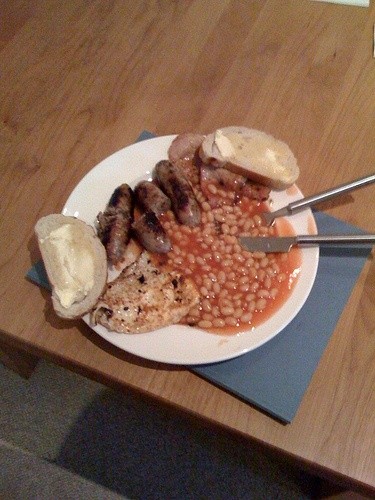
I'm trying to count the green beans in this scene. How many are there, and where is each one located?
[163,183,288,329]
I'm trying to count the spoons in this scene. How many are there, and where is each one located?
[263,173,375,226]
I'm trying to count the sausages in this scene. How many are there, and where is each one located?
[101,160,200,263]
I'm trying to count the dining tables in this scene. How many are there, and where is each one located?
[1,0,375,491]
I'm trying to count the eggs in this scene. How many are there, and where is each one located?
[90,250,200,335]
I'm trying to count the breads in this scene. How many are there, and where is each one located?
[35,213,108,319]
[200,126,299,189]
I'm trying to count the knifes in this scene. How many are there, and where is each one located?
[238,231,375,253]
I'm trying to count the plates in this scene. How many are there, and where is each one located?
[60,135,321,365]
